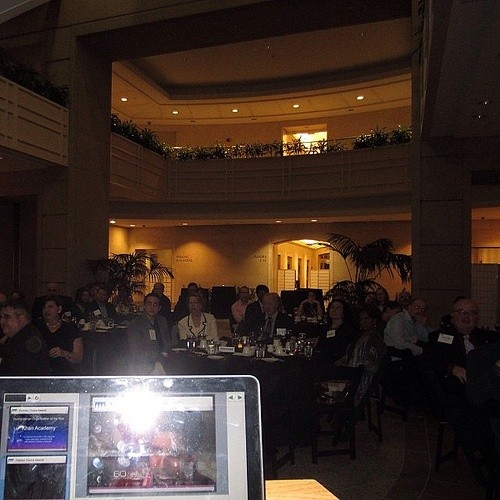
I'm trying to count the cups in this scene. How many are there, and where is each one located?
[208,344,215,355]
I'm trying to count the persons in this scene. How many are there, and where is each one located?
[146,414,215,487]
[430,298,500,500]
[0,282,463,453]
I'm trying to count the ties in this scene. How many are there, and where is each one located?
[411,320,419,343]
[265,316,272,336]
[463,334,475,355]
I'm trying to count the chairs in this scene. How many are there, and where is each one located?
[210,286,236,321]
[280,288,325,327]
[419,348,460,473]
[275,327,389,465]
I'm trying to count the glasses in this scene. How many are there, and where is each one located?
[258,290,267,295]
[412,306,427,312]
[376,292,386,297]
[0,313,22,322]
[453,308,479,315]
[146,301,158,308]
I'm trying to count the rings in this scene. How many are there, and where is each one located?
[56,353,59,355]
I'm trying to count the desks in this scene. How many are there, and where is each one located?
[265,479,339,500]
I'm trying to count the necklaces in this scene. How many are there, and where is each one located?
[49,320,59,329]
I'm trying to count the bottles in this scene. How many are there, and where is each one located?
[267,339,287,355]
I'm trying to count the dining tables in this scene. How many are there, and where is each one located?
[172,340,322,443]
[68,319,128,375]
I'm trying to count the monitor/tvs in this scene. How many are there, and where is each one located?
[0,375,266,500]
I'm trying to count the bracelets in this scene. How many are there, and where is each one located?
[453,367,458,375]
[63,351,67,357]
